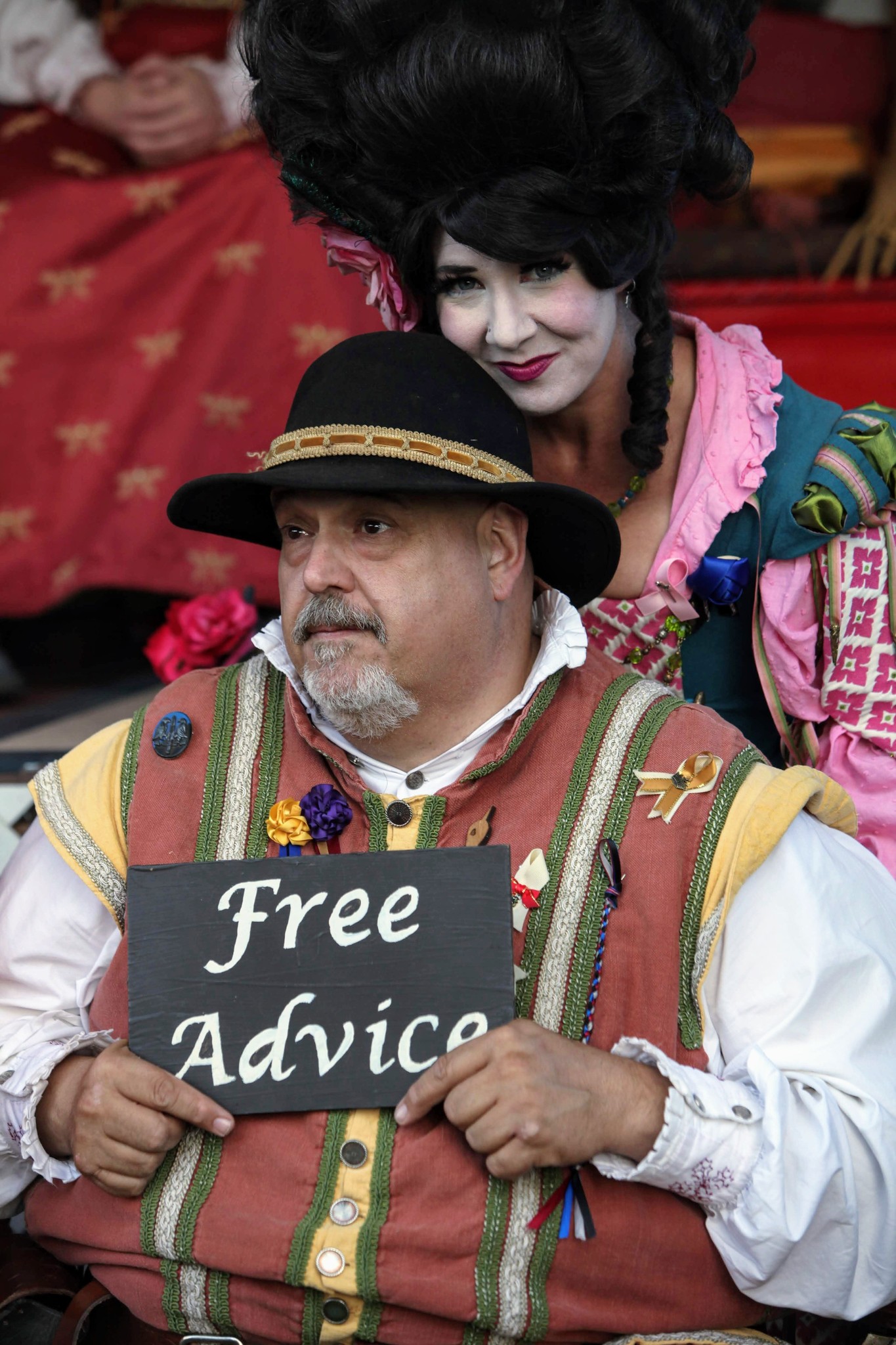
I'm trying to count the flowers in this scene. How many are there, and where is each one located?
[144,588,263,684]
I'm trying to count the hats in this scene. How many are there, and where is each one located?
[165,332,622,610]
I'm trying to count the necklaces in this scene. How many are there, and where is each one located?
[607,474,644,516]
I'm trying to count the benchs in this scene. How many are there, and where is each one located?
[658,13,896,419]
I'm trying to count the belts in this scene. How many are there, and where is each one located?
[55,1282,240,1345]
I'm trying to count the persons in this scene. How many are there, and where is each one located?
[0,330,895,1344]
[239,0,896,911]
[0,0,387,698]
